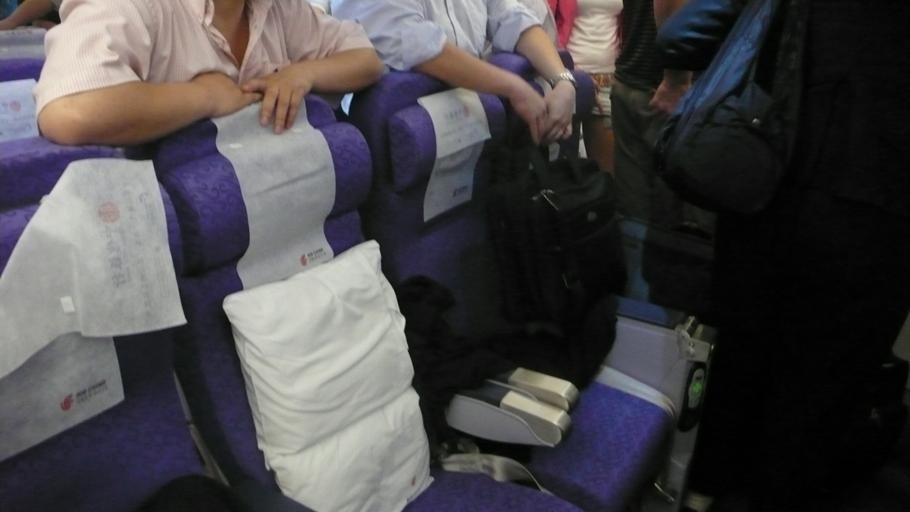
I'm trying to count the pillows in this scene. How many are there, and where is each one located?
[220,239,417,471]
[271,389,435,512]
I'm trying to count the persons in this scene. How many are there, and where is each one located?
[322,1,577,149]
[1,1,68,33]
[542,1,909,511]
[33,1,387,145]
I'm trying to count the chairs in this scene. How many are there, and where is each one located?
[128,96,582,512]
[0,137,230,512]
[0,27,49,120]
[348,70,681,512]
[490,46,595,154]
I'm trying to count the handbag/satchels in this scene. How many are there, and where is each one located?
[646,0,807,215]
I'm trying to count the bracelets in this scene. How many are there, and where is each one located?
[548,68,577,88]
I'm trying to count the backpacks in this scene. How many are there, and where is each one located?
[488,132,619,392]
